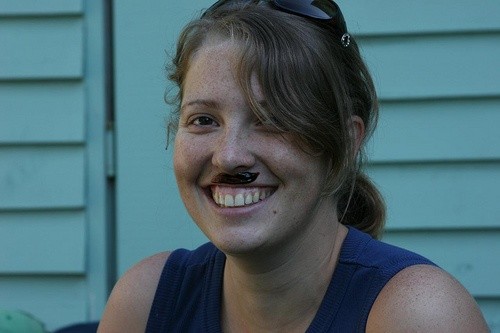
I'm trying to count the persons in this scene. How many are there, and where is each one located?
[95,2,494,332]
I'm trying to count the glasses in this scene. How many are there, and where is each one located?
[200,0,353,51]
